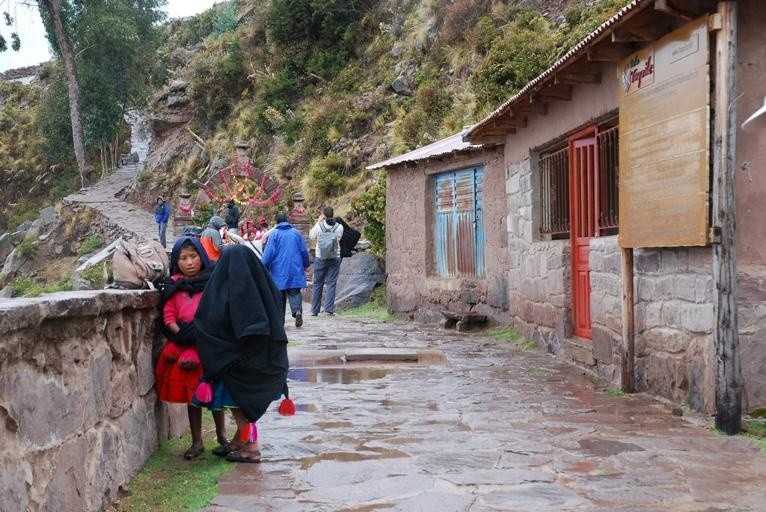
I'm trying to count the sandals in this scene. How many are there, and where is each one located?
[214,435,261,463]
[183,442,205,460]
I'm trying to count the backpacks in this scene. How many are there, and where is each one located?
[318,220,339,259]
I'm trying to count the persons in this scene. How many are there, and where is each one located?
[200,211,310,327]
[309,207,344,316]
[225,197,240,228]
[154,235,260,464]
[155,196,169,248]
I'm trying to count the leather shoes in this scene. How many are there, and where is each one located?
[312,311,335,316]
[295,311,303,327]
